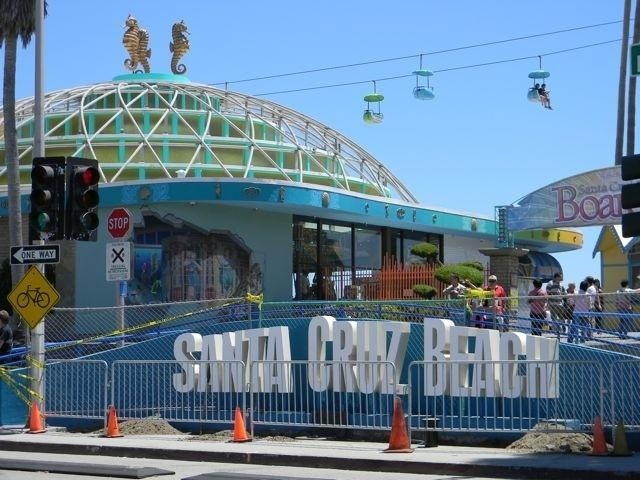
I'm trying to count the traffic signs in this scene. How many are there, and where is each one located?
[10,244,60,265]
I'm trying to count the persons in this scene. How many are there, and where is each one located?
[615,280,640,339]
[441,273,604,344]
[301,272,310,299]
[0,309,14,364]
[540,84,553,110]
[535,83,545,105]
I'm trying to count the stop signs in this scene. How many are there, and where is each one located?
[107,208,131,239]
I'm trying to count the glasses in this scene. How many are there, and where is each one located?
[489,279,496,283]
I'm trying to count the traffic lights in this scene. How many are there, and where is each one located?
[68,156,99,239]
[30,156,66,236]
[621,154,640,239]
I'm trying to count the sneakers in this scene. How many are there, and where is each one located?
[543,104,555,113]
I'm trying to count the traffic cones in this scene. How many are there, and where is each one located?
[98,405,124,438]
[228,406,253,444]
[609,419,635,458]
[25,401,48,435]
[380,396,416,454]
[583,414,612,457]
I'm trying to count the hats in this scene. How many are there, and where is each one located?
[487,275,498,280]
